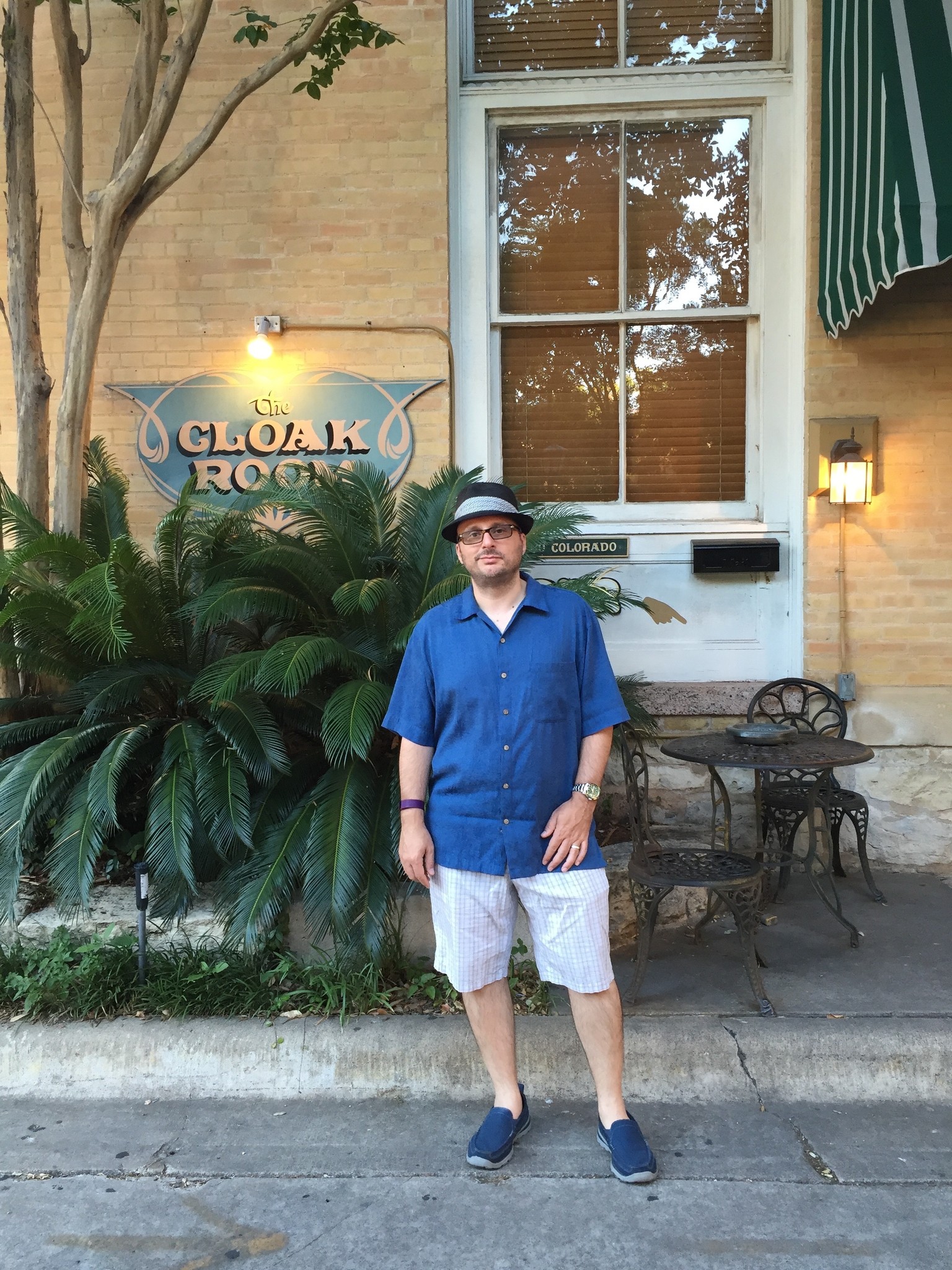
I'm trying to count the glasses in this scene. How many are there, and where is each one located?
[458,524,522,545]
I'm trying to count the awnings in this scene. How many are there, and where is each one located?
[817,0,952,340]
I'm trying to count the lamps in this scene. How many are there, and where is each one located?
[829,427,873,505]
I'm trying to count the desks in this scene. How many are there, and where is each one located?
[660,733,874,948]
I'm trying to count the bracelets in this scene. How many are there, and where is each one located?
[398,799,424,810]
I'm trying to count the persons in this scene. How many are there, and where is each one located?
[379,482,658,1184]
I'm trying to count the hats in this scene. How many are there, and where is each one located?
[441,481,534,543]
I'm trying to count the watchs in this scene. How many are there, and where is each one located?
[572,783,601,800]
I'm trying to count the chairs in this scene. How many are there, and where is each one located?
[623,677,887,1017]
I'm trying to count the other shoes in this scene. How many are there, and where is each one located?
[596,1109,657,1183]
[466,1083,531,1170]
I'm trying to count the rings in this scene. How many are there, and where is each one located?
[571,846,580,849]
